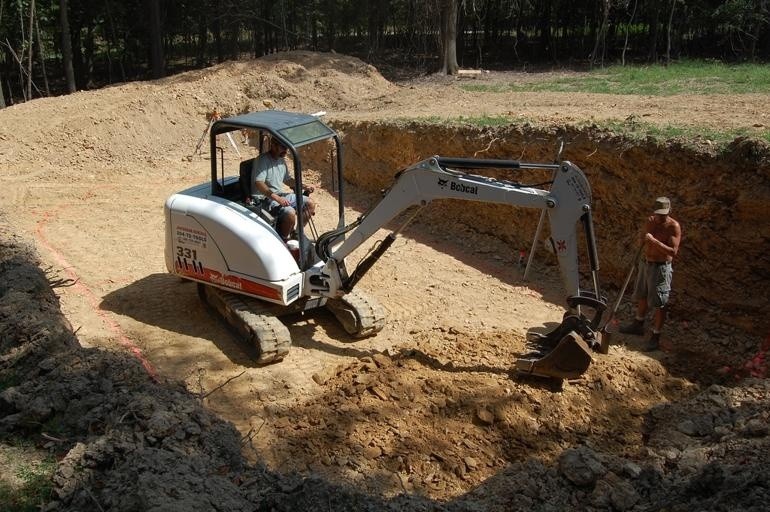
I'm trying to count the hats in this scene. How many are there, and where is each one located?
[653,197,671,216]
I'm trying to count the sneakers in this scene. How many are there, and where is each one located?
[618,319,645,336]
[641,331,661,352]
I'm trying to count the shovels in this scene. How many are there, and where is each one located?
[599,246,643,355]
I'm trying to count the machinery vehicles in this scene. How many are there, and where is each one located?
[164,111,608,380]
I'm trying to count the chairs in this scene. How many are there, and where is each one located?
[238,158,254,202]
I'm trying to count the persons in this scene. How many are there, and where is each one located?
[619,198,682,353]
[251,133,315,243]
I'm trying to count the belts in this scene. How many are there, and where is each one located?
[640,257,671,267]
